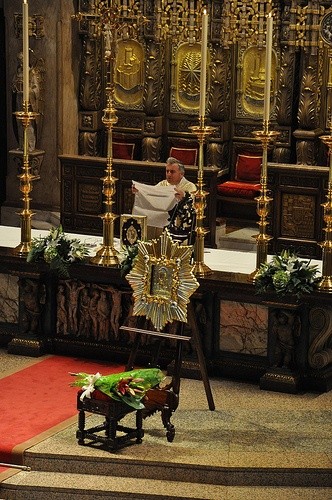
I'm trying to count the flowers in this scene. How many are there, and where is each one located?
[251,249,320,302]
[67,368,173,419]
[26,224,89,278]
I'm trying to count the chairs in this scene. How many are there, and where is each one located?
[112,142,135,160]
[170,147,197,166]
[217,154,262,203]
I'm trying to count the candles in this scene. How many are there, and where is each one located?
[22,0,30,101]
[264,13,274,119]
[199,10,208,116]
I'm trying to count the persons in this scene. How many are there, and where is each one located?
[131,157,196,226]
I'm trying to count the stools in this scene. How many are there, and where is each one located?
[77,393,179,454]
